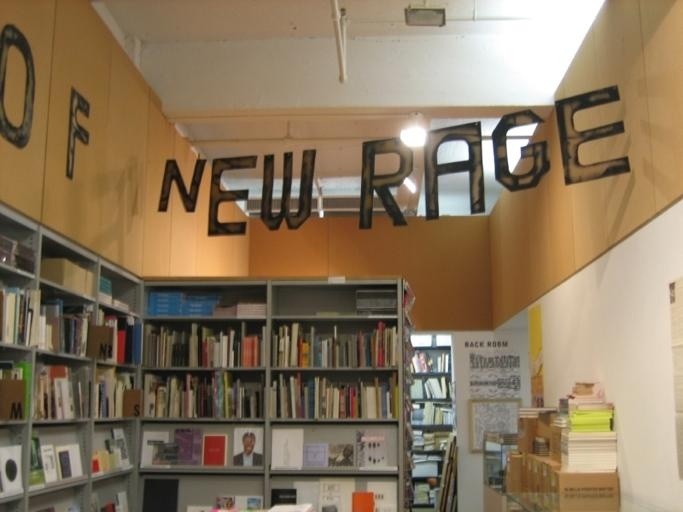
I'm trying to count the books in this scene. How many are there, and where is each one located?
[2,427,129,512]
[402,281,457,512]
[507,381,619,512]
[271,427,397,511]
[140,319,399,421]
[0,285,141,424]
[140,428,265,511]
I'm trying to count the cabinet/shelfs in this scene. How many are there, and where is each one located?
[142,279,406,512]
[0,200,142,511]
[410,346,452,512]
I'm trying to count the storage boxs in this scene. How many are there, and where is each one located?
[507,416,620,511]
